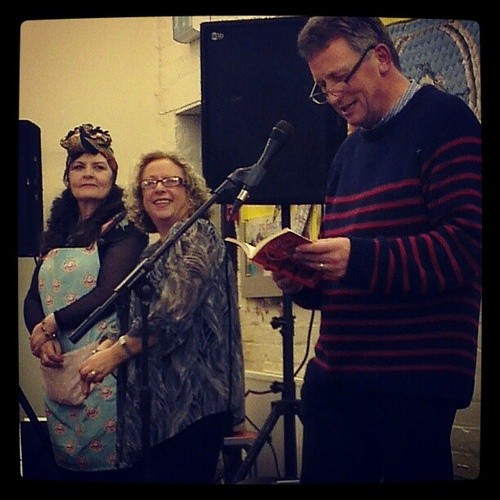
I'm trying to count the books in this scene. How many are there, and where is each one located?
[221,227,323,291]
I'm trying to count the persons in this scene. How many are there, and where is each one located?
[79,149,244,484]
[272,16,484,480]
[24,120,150,479]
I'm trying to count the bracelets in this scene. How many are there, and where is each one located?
[92,350,101,355]
[42,319,57,340]
[119,336,135,360]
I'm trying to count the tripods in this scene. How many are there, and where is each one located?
[227,202,310,485]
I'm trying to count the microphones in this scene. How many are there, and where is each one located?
[230,120,295,215]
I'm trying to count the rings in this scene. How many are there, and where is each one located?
[42,360,45,365]
[320,264,325,273]
[92,371,97,377]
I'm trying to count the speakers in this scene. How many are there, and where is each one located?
[200,16,350,202]
[17,120,44,259]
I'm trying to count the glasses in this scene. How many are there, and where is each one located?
[312,45,382,106]
[140,176,187,190]
